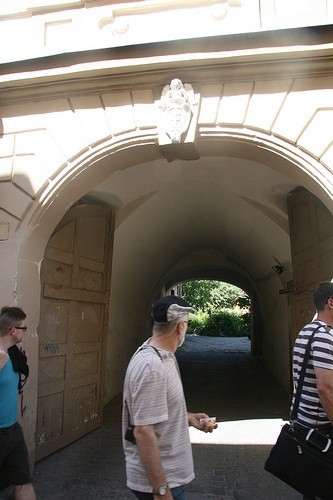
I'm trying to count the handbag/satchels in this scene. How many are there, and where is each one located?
[264,423,333,500]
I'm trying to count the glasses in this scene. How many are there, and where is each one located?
[9,326,27,331]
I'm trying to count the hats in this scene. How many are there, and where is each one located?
[150,296,196,322]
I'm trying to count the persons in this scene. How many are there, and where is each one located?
[0,307,36,500]
[122,294,218,500]
[290,281,333,500]
[154,79,198,142]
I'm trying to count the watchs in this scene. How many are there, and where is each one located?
[153,482,169,496]
